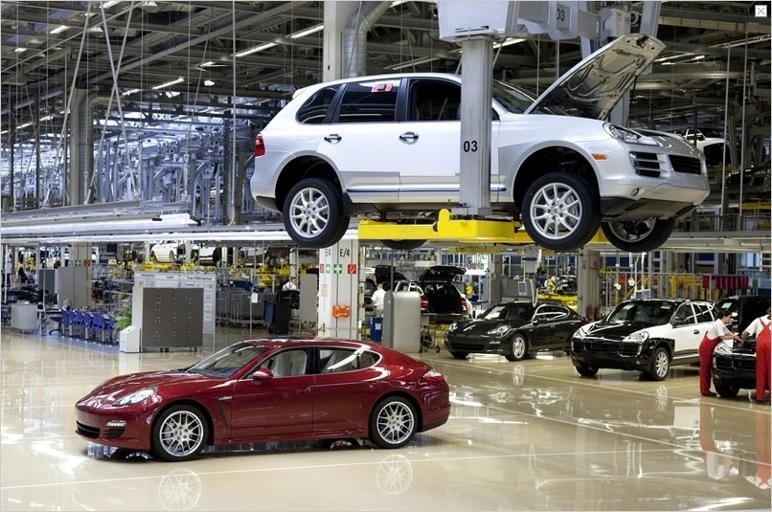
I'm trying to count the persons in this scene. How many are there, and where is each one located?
[18,266,28,283]
[740,306,771,406]
[0,268,11,288]
[696,310,743,397]
[370,283,386,317]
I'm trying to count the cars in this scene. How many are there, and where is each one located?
[71,336,453,462]
[362,262,477,333]
[443,301,588,363]
[568,295,724,381]
[710,294,772,400]
[247,69,710,259]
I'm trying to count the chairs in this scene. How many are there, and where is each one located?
[271,351,306,377]
[48,306,117,347]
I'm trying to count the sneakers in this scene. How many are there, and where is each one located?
[756,399,771,405]
[702,391,717,398]
